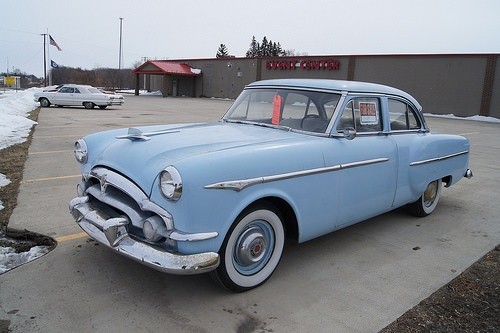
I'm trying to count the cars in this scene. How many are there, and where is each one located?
[43,85,65,92]
[33,85,125,110]
[69,78,473,294]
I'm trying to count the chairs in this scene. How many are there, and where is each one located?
[284,116,405,133]
